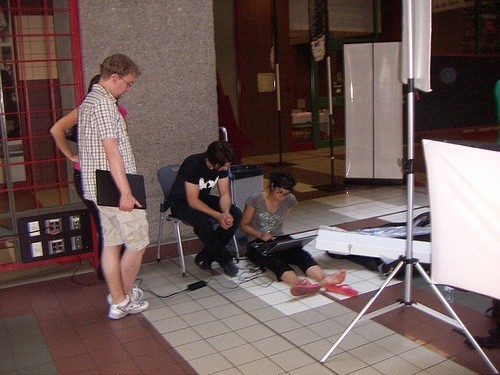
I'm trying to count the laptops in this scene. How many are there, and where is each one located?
[251,233,318,256]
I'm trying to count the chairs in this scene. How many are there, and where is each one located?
[156,164,240,278]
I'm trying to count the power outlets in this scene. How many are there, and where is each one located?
[46,213,83,255]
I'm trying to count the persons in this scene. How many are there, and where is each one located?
[50,74,128,280]
[239,172,346,296]
[79,53,150,319]
[169,140,243,276]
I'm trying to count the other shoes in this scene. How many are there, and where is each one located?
[221,261,240,276]
[194,256,218,275]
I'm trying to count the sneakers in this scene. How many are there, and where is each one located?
[105,288,150,320]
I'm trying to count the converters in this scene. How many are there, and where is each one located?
[188,280,206,290]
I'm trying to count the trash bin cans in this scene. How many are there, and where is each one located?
[230,163,263,240]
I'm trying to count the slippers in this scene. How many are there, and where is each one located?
[325,283,359,297]
[289,279,321,296]
